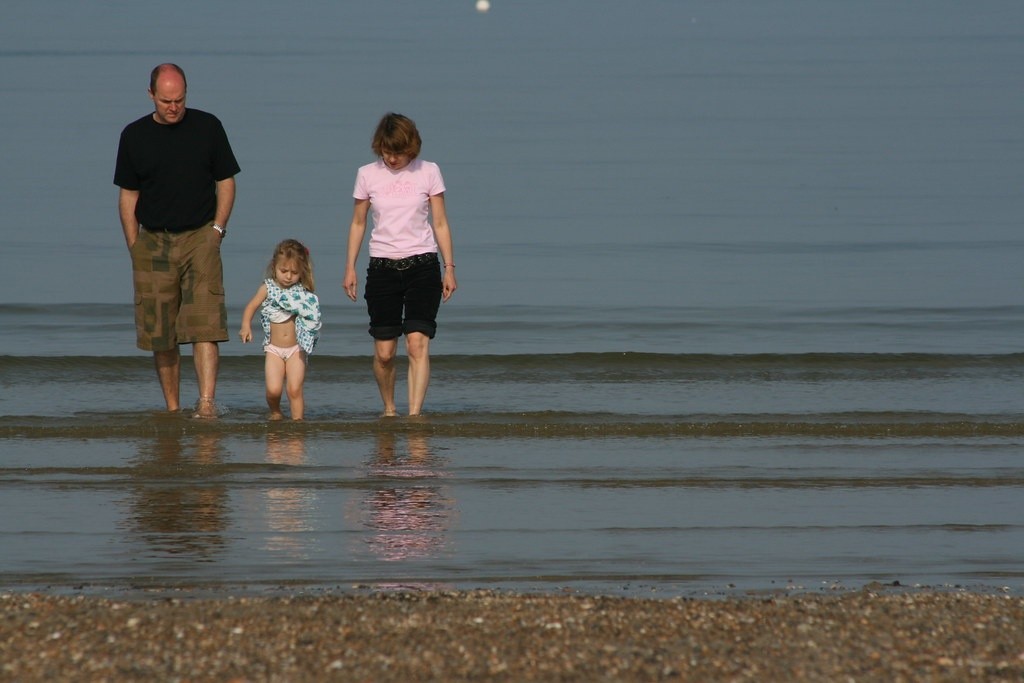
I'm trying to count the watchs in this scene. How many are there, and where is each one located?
[443,263,455,268]
[210,223,226,238]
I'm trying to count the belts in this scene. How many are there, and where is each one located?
[370,252,436,270]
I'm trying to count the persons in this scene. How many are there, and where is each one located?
[343,113,456,416]
[113,63,242,419]
[238,239,322,420]
